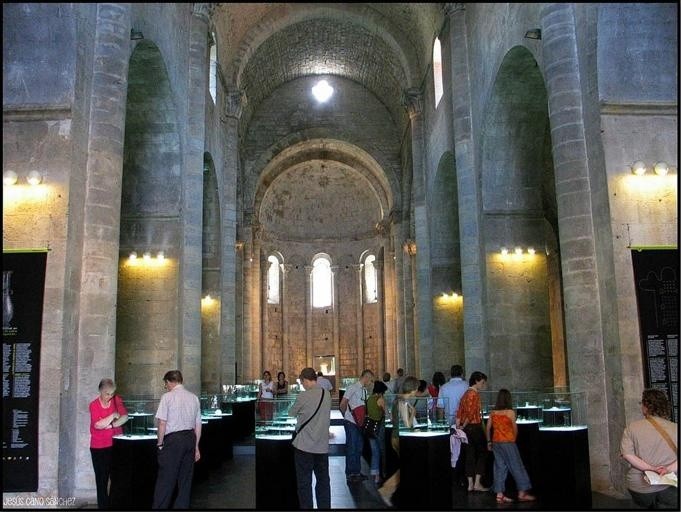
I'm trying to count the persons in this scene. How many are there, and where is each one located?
[151,370,202,510]
[253,364,537,512]
[87,377,129,511]
[619,388,679,509]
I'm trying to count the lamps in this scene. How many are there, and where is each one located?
[500,247,536,257]
[128,251,164,260]
[631,160,672,180]
[2,169,44,186]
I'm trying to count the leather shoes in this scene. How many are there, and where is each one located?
[348,473,392,507]
[468,487,491,492]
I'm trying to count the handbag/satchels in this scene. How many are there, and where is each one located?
[361,418,381,438]
[466,425,488,457]
[351,405,367,428]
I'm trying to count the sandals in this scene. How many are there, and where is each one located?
[496,496,513,504]
[518,494,535,501]
